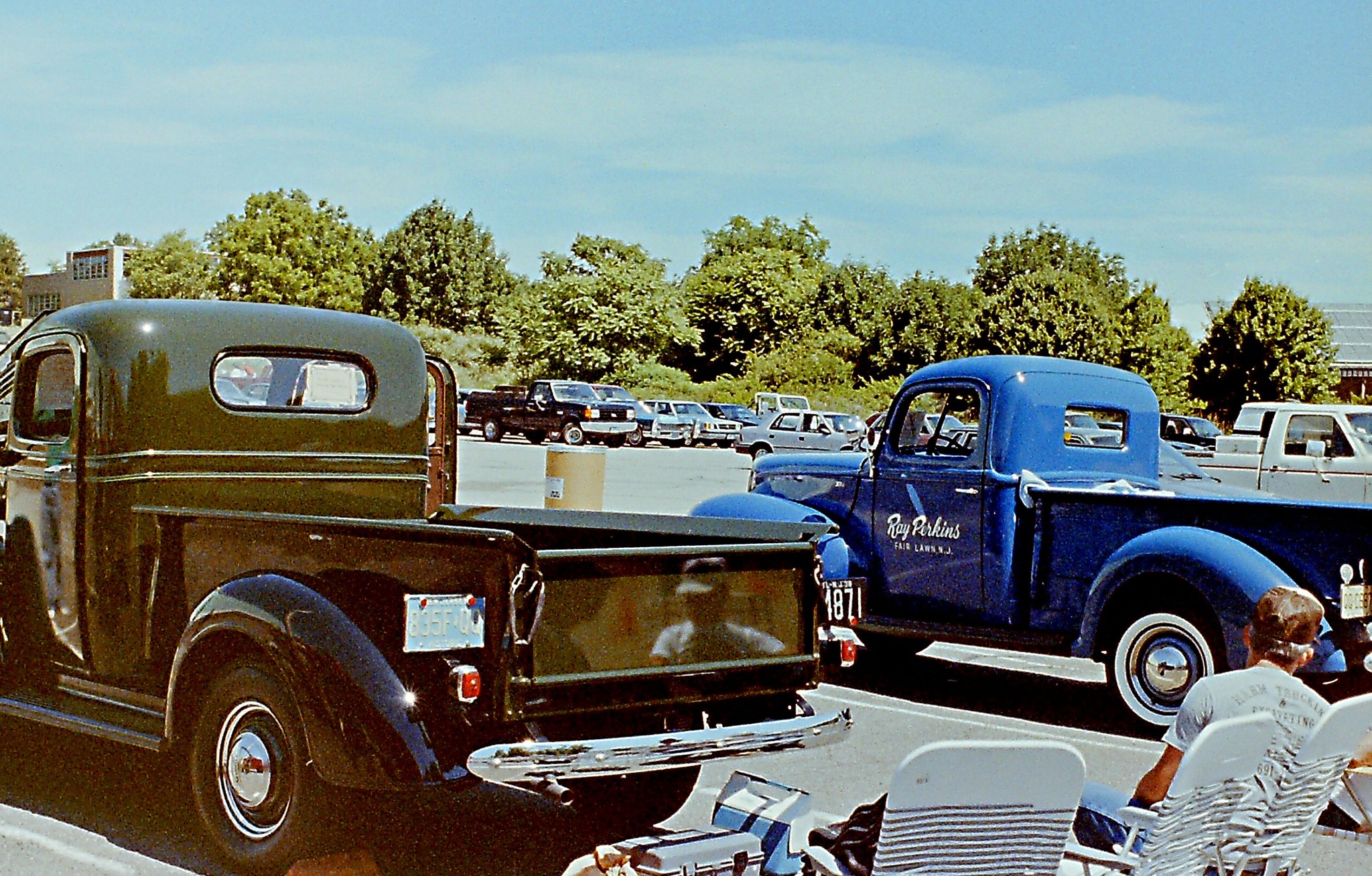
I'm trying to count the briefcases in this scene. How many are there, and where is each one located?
[613,826,765,876]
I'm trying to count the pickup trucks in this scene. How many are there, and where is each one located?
[430,378,830,449]
[1064,400,1372,504]
[0,298,858,876]
[689,353,1372,744]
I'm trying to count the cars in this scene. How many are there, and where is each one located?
[865,410,980,457]
[733,409,869,463]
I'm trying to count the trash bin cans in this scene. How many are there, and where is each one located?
[543,445,606,512]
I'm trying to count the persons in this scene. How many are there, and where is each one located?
[1071,585,1324,876]
[649,558,783,666]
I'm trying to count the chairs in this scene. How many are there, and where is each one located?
[806,692,1371,876]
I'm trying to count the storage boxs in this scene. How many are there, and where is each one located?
[612,824,764,876]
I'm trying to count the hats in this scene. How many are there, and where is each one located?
[676,556,729,595]
[1252,586,1324,644]
